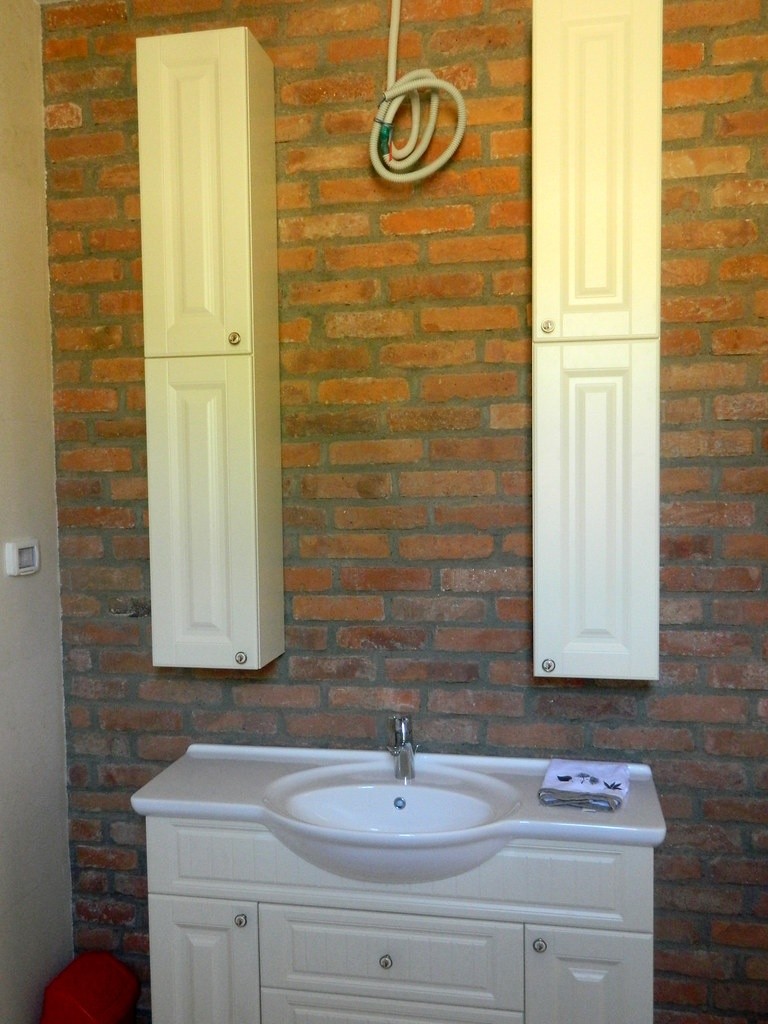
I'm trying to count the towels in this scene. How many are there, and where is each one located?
[537,758,632,813]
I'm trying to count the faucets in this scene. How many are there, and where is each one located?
[385,710,422,780]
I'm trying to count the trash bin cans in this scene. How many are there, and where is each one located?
[40,949,141,1024]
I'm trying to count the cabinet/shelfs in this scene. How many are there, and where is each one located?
[143,815,655,1024]
[531,0,658,682]
[136,26,284,670]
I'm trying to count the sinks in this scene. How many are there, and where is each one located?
[267,761,522,883]
